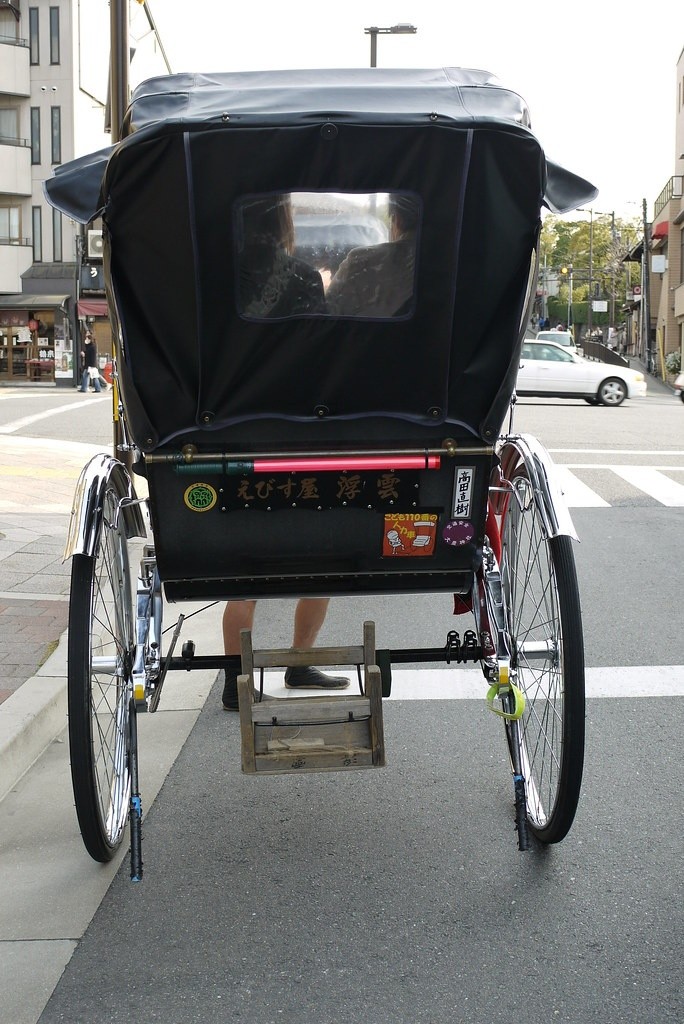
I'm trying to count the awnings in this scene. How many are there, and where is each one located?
[78,298,108,316]
[621,239,644,263]
[0,294,72,314]
[651,221,669,240]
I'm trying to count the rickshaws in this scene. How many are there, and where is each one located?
[40,66,586,884]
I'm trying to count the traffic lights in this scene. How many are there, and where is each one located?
[555,269,574,274]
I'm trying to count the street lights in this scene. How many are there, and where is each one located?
[364,23,419,215]
[576,209,592,331]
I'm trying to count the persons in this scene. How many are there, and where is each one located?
[539,317,550,331]
[556,323,563,331]
[567,326,572,331]
[611,327,619,351]
[222,598,350,711]
[326,194,416,317]
[76,330,113,393]
[595,327,602,335]
[239,193,328,314]
[532,314,537,329]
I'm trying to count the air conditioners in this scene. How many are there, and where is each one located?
[88,230,103,257]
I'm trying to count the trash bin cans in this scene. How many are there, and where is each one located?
[104,362,112,383]
[588,335,603,344]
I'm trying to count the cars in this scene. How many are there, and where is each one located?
[536,330,581,356]
[514,340,648,407]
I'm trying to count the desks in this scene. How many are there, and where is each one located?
[24,359,55,382]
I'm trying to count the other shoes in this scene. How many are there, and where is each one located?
[285,667,351,690]
[78,390,86,392]
[92,390,100,393]
[221,689,273,711]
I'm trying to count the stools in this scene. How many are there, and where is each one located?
[236,621,387,776]
